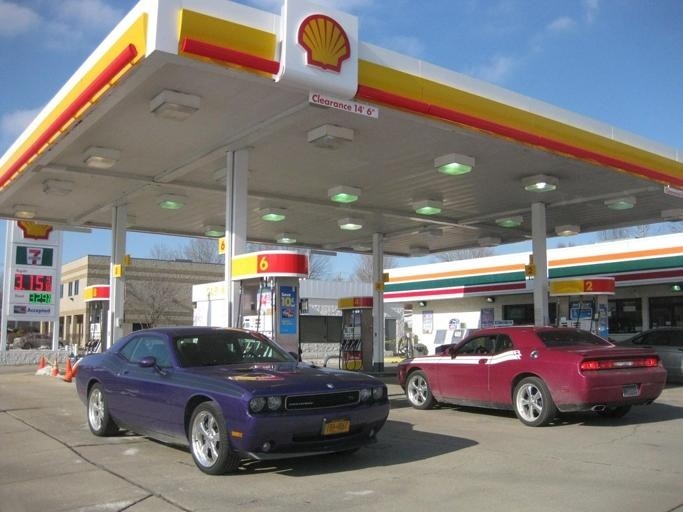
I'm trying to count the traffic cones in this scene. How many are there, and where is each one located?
[35,355,81,382]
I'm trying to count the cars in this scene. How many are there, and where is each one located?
[12,332,65,350]
[612,326,682,387]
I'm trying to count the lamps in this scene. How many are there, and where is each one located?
[476,216,524,247]
[433,151,476,175]
[521,174,559,193]
[148,89,203,123]
[155,186,444,245]
[9,180,72,219]
[555,196,636,236]
[306,124,354,150]
[81,146,121,169]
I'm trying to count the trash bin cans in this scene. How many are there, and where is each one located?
[414,343,426,356]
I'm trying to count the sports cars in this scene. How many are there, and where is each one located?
[394,322,668,427]
[74,324,391,476]
